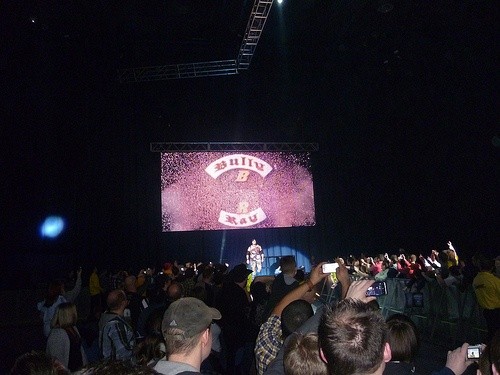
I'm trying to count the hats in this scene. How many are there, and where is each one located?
[162,297,222,338]
[229,264,253,282]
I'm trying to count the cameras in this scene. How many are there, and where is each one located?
[465,346,482,361]
[365,280,388,297]
[321,262,339,274]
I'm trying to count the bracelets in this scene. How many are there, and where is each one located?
[305,278,314,293]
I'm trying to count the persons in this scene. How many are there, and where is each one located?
[139,312,174,369]
[0,245,500,342]
[46,302,89,375]
[382,317,419,375]
[11,351,71,375]
[245,238,264,283]
[255,260,351,375]
[263,278,375,375]
[145,298,222,375]
[97,289,143,365]
[317,298,392,375]
[431,331,500,375]
